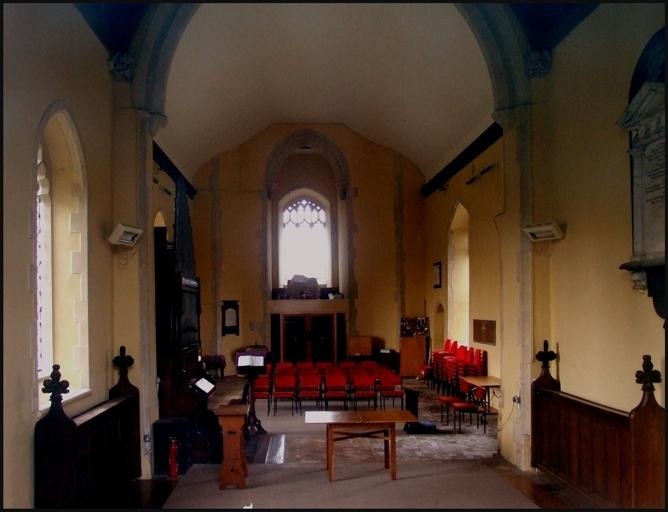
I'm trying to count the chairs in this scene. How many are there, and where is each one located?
[255,361,404,416]
[432,339,488,434]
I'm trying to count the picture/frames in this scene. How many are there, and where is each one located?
[431,261,442,288]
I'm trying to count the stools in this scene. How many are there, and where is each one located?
[202,354,225,379]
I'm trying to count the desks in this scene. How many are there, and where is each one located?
[458,375,501,428]
[216,404,250,489]
[303,407,418,482]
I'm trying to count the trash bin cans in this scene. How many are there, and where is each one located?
[152,418,193,476]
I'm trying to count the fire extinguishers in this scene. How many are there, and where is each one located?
[168,436,180,478]
